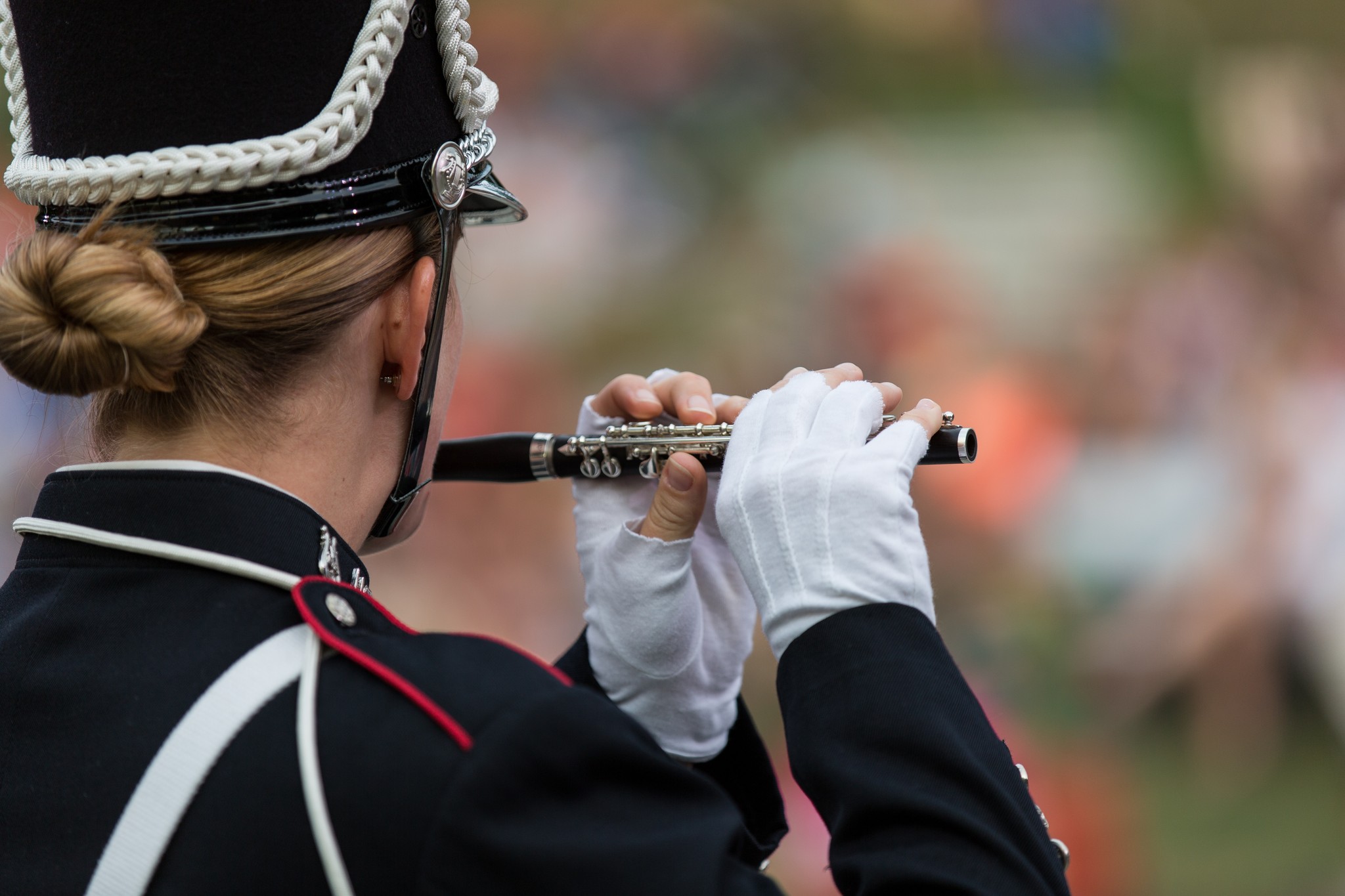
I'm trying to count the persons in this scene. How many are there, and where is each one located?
[0,0,1070,895]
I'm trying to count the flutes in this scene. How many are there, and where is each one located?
[432,411,982,488]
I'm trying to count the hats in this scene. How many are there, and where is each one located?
[1,1,532,246]
